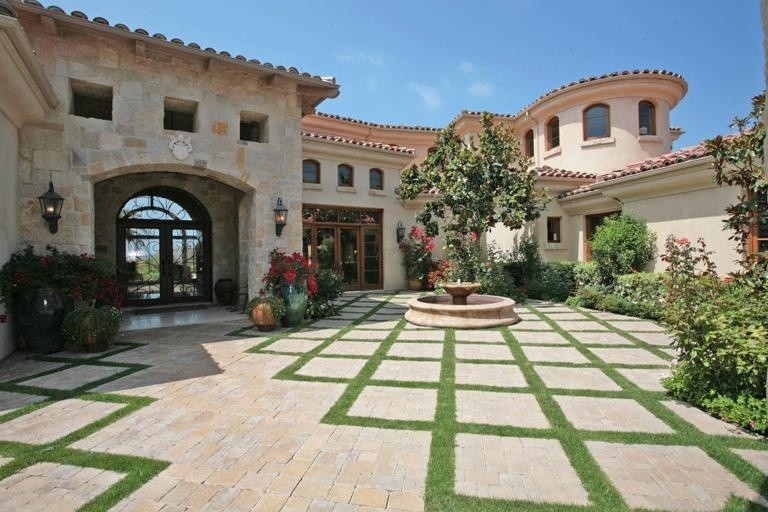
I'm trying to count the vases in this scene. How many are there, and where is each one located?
[409,278,424,291]
[13,289,74,354]
[278,292,309,327]
[214,278,235,304]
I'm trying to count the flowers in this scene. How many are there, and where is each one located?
[0,242,126,324]
[400,226,435,281]
[261,247,322,300]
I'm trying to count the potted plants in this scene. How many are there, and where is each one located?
[244,296,287,332]
[60,304,126,354]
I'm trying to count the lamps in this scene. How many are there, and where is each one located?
[272,196,289,237]
[395,221,407,243]
[37,181,66,234]
